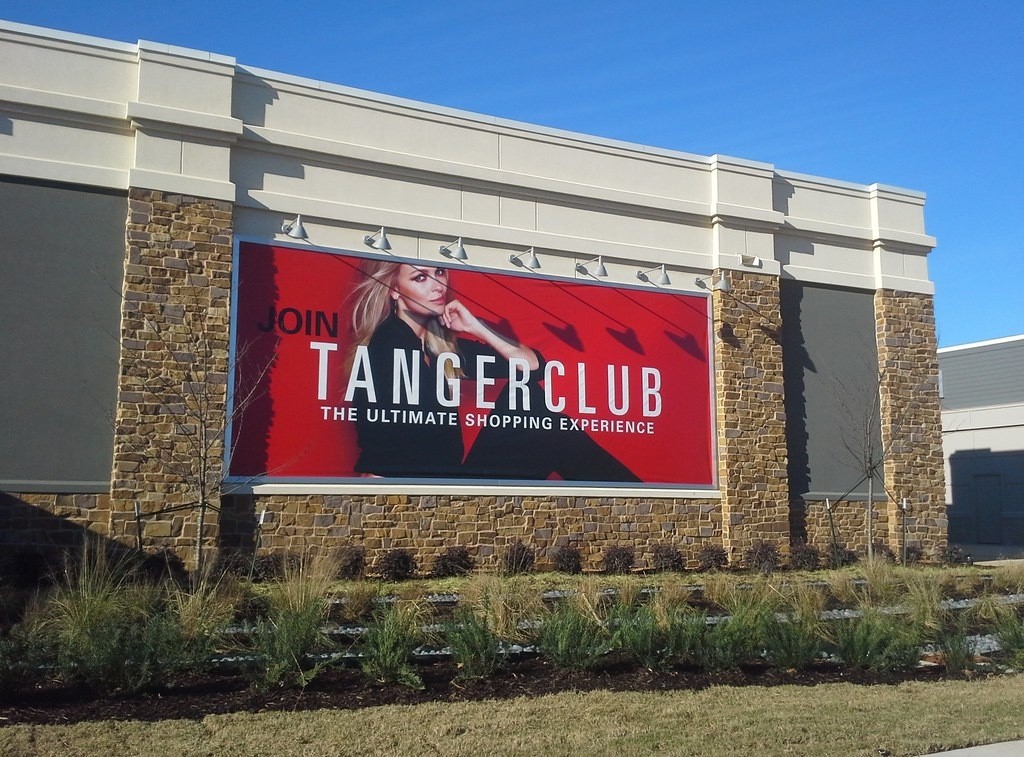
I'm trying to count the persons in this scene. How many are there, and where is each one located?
[348,260,642,482]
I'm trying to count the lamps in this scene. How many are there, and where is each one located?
[574,255,609,276]
[508,247,541,269]
[693,269,730,292]
[439,236,468,260]
[281,214,309,239]
[363,225,392,249]
[636,263,671,285]
